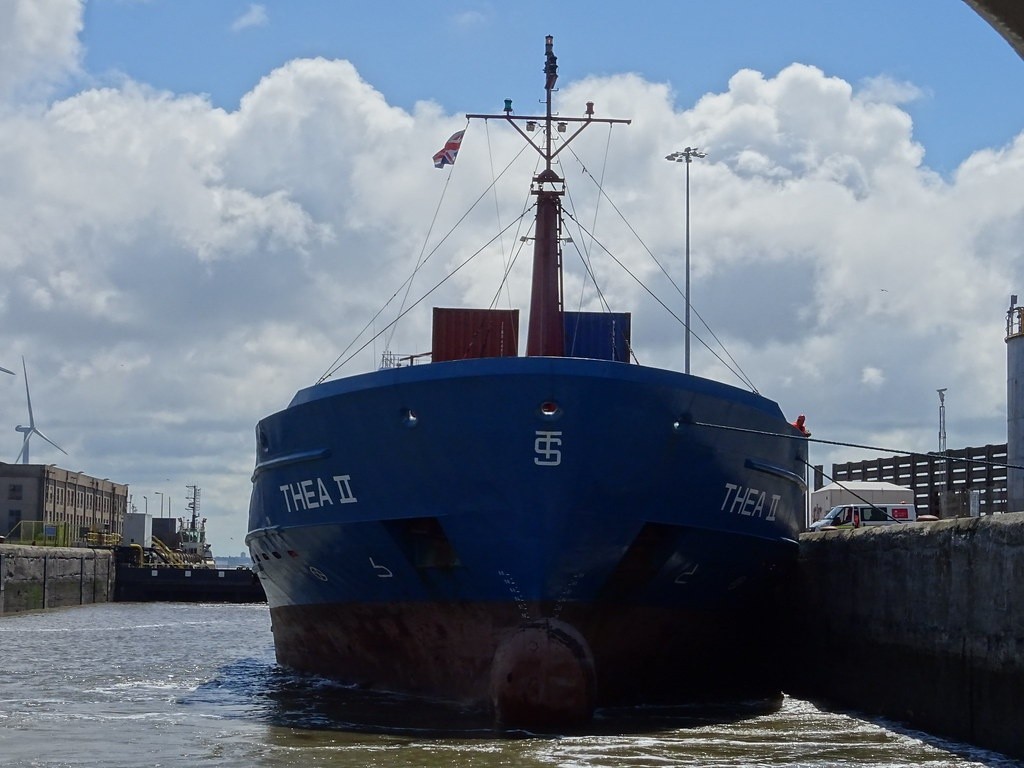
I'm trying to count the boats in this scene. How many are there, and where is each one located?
[242,32,810,731]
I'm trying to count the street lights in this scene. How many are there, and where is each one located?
[665,146,710,377]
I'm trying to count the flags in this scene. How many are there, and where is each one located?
[432,130,463,168]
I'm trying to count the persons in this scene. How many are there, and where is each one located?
[846,510,859,527]
[791,414,812,439]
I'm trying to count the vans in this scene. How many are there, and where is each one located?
[810,502,921,531]
